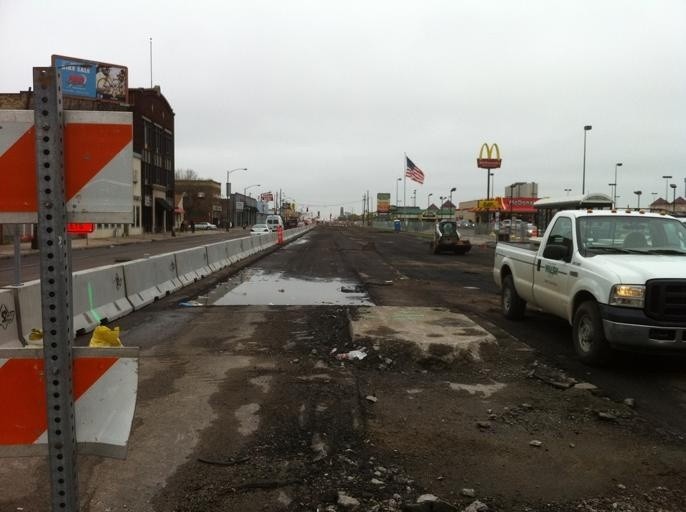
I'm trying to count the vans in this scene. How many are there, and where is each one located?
[285,216,299,229]
[265,214,284,232]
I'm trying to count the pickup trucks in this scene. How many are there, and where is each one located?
[493,208,686,367]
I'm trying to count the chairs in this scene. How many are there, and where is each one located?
[623,231,649,250]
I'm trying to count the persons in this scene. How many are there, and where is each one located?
[225,223,229,232]
[191,220,195,233]
[243,224,246,229]
[180,221,184,232]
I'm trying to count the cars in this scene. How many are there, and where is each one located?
[249,223,272,237]
[187,221,217,230]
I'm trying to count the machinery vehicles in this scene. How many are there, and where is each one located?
[430,219,473,256]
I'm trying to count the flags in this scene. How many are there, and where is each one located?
[406,157,425,185]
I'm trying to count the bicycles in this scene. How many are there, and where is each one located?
[98,68,124,98]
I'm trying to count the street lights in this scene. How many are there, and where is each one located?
[582,125,592,196]
[670,183,677,214]
[449,188,456,220]
[244,184,260,201]
[633,191,642,208]
[427,193,433,217]
[225,167,248,232]
[440,196,444,221]
[613,162,623,209]
